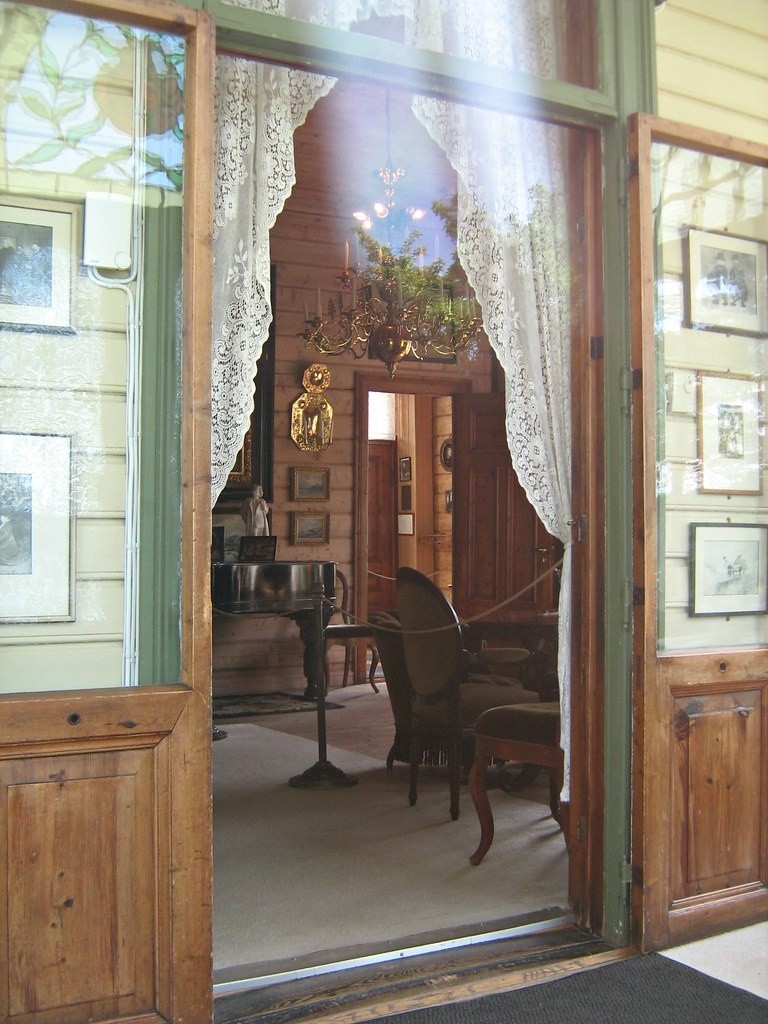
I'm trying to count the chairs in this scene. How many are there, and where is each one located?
[371,611,527,769]
[394,566,539,821]
[469,702,571,866]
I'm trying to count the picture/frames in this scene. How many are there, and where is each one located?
[290,467,331,502]
[239,536,278,563]
[689,523,768,617]
[398,512,414,536]
[0,430,78,625]
[211,526,226,563]
[697,369,764,495]
[682,222,768,339]
[290,512,330,546]
[212,507,273,564]
[211,263,276,504]
[0,186,86,334]
[401,485,412,511]
[399,457,411,482]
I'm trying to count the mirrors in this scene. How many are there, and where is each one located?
[445,490,453,514]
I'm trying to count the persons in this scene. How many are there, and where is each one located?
[707,252,748,307]
[719,411,743,456]
[0,509,29,566]
[242,484,269,536]
[0,236,49,300]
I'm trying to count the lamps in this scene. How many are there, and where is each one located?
[295,88,485,381]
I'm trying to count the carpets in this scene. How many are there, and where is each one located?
[213,689,345,719]
[351,947,768,1024]
